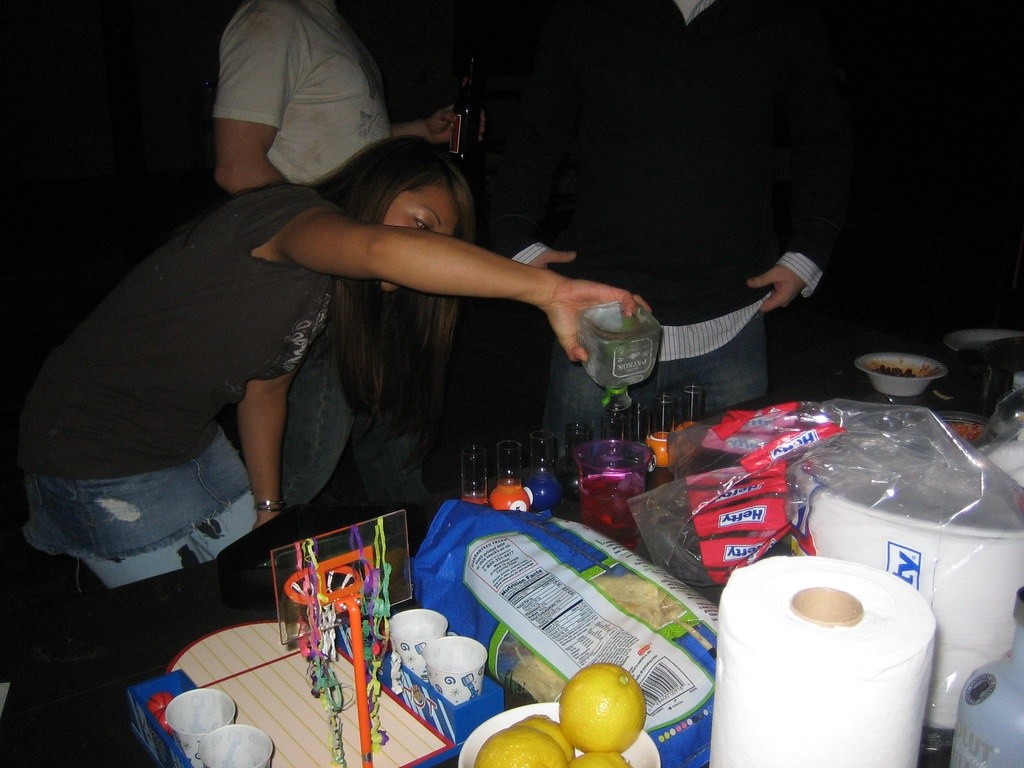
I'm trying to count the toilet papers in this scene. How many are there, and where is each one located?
[793,447,1024,730]
[710,553,937,768]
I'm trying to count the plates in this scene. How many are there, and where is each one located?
[944,329,1024,351]
[458,702,661,768]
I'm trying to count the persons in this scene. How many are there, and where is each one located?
[488,0,856,454]
[15,134,652,591]
[210,0,485,513]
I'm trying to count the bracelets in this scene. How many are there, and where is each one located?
[254,499,287,512]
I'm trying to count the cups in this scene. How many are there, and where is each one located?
[422,635,488,706]
[389,608,449,683]
[165,688,236,768]
[572,441,653,550]
[199,724,274,768]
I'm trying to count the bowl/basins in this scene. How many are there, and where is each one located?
[855,352,948,397]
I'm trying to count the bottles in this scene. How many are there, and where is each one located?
[449,57,481,170]
[578,299,662,412]
[949,586,1024,768]
[460,383,706,513]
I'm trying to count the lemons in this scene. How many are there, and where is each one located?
[559,664,647,755]
[473,727,567,768]
[566,750,633,768]
[508,715,575,762]
